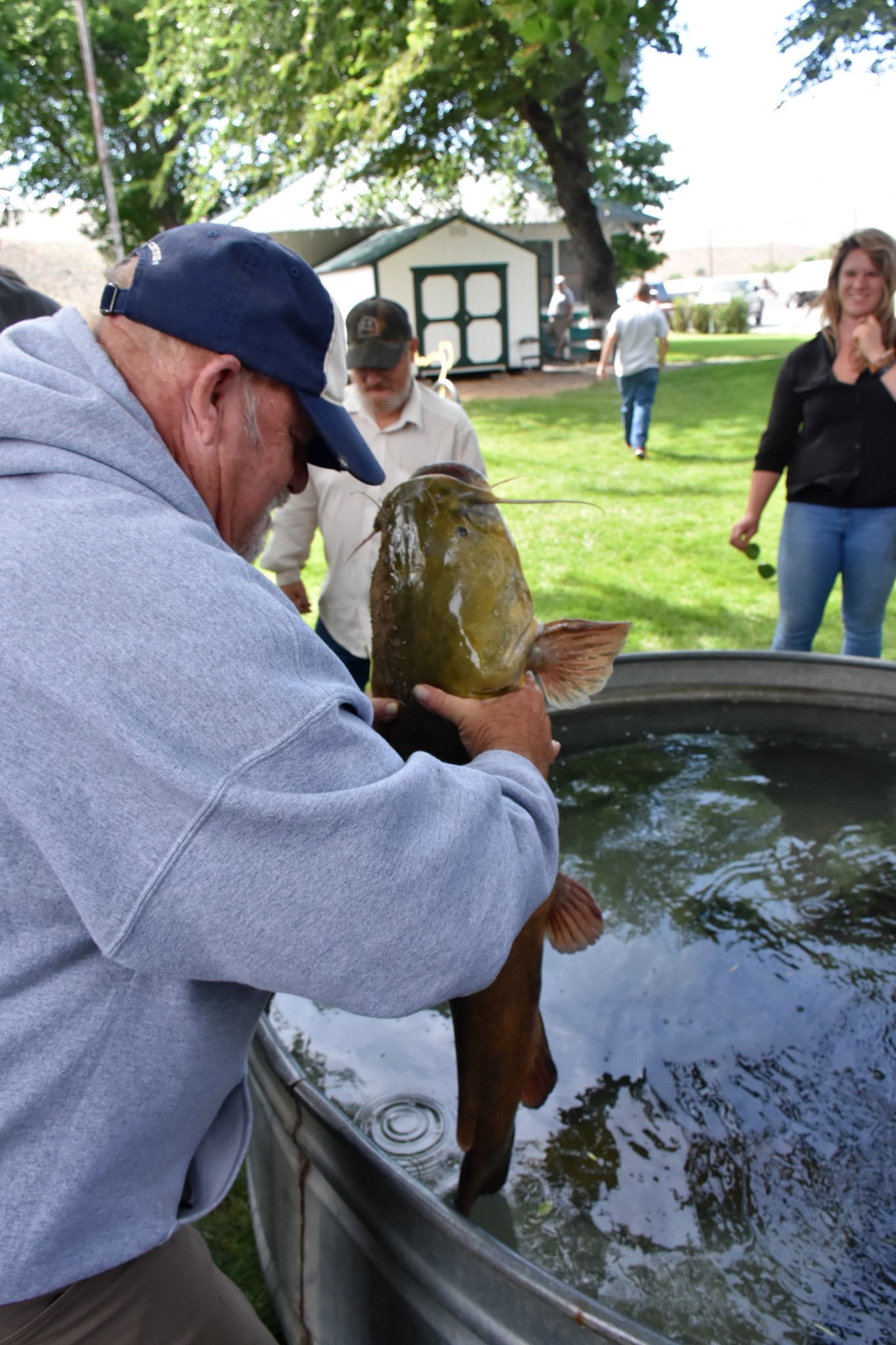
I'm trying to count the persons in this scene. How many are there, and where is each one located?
[545,275,577,361]
[596,282,672,460]
[729,228,896,659]
[0,220,563,1345]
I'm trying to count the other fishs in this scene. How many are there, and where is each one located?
[350,462,634,1216]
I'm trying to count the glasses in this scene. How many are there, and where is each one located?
[744,544,775,579]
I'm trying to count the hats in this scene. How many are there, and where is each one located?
[555,276,565,284]
[346,296,412,369]
[101,222,386,485]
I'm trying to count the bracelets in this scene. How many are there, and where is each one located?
[869,348,895,379]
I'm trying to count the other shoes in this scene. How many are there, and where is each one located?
[634,447,645,459]
